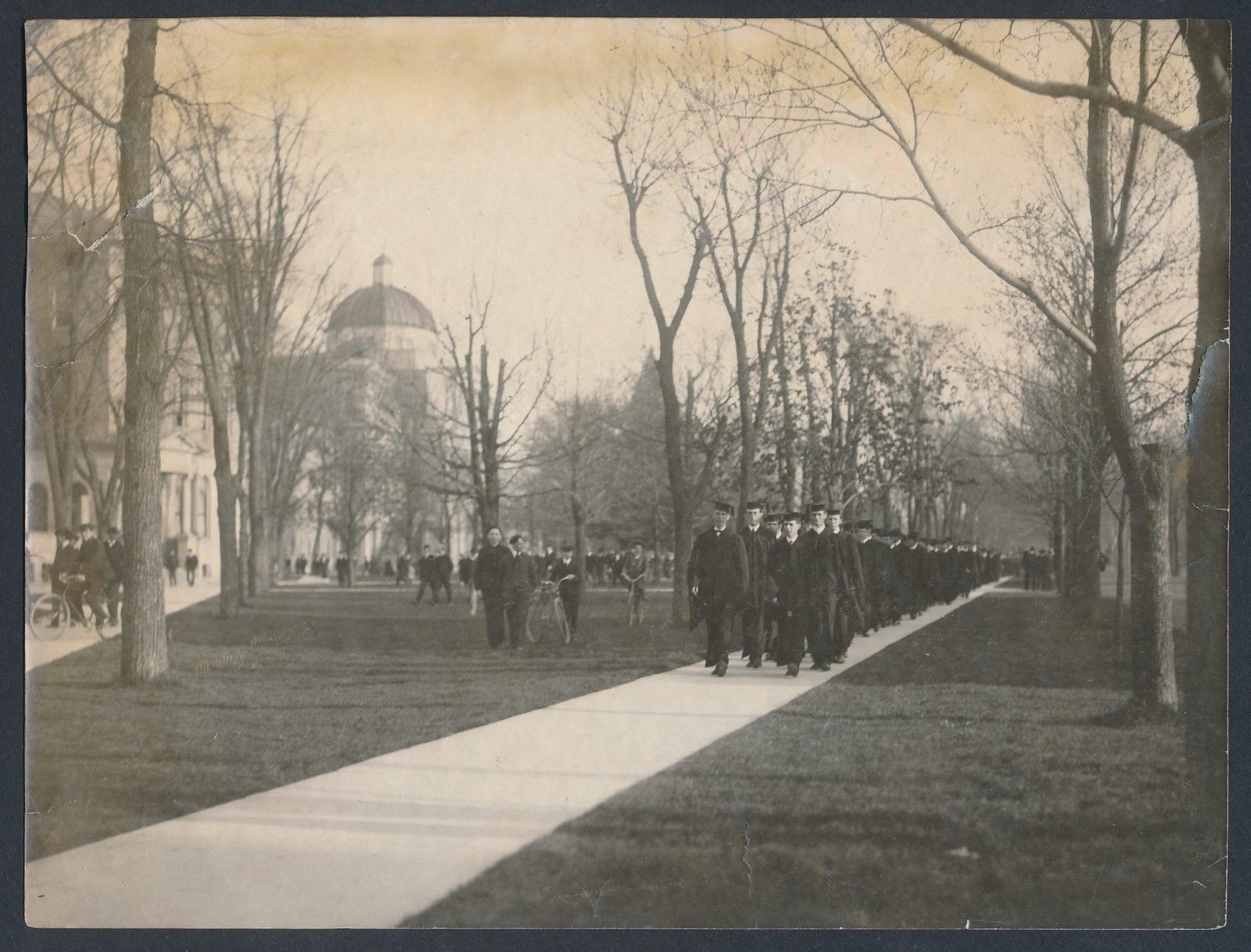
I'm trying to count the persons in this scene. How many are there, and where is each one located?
[26,523,197,630]
[284,501,1059,675]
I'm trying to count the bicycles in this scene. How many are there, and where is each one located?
[525,575,575,643]
[619,571,645,626]
[30,574,122,642]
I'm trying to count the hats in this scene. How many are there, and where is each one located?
[708,498,1001,561]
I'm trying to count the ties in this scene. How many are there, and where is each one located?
[515,552,518,558]
[565,560,567,566]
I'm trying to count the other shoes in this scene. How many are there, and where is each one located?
[700,579,1000,677]
